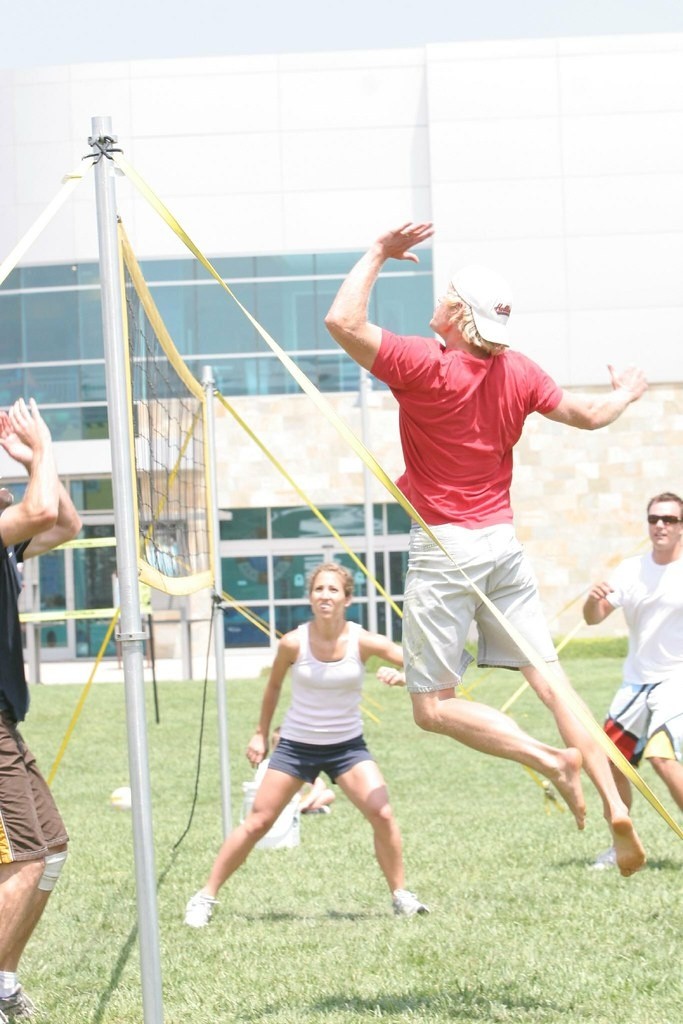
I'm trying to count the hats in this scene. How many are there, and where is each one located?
[455,259,514,347]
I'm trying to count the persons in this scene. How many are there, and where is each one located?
[255,727,335,814]
[324,222,649,877]
[183,563,429,928]
[584,492,683,873]
[0,398,83,1024]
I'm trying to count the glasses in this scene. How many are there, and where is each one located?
[648,513,683,524]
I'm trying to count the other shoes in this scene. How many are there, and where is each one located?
[305,806,331,815]
[588,847,618,869]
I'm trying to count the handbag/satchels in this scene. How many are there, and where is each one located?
[241,760,300,851]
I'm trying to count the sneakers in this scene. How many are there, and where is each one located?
[393,888,429,917]
[0,983,46,1024]
[183,889,221,926]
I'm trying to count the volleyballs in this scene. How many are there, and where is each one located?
[111,787,132,809]
